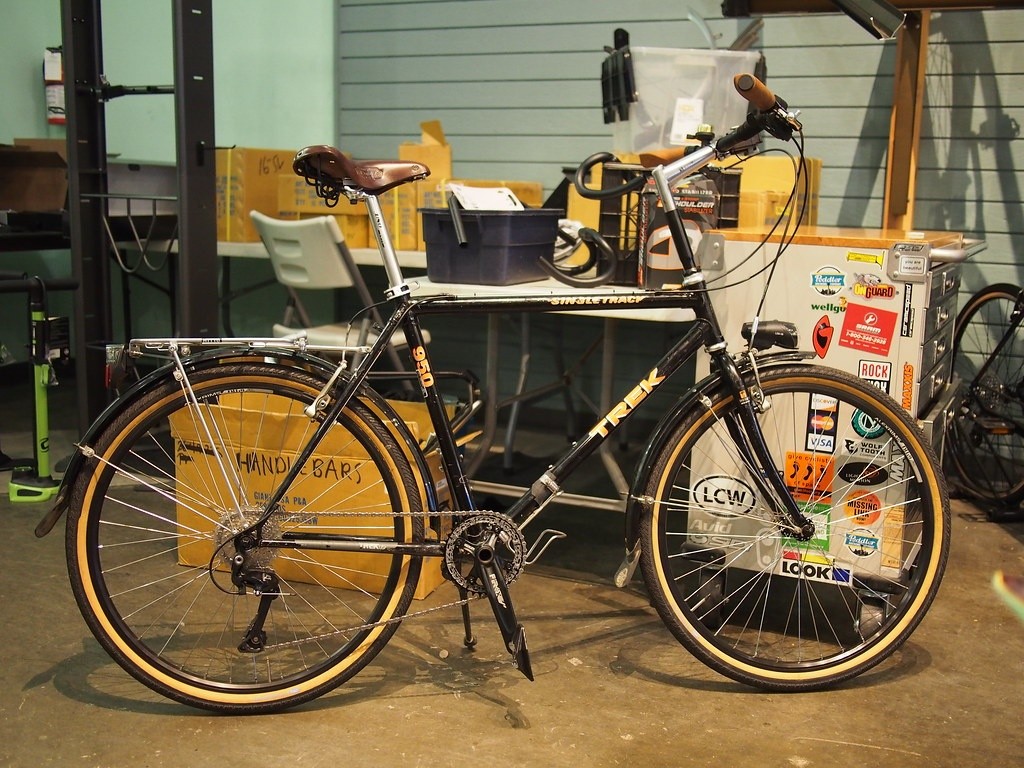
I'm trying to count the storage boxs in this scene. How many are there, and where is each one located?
[168,394,457,601]
[217,126,821,285]
[0,139,67,215]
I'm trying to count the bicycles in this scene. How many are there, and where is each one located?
[32,67,953,712]
[950,281,1024,509]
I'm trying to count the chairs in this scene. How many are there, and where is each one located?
[250,211,431,393]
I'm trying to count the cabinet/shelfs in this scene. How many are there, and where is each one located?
[686,228,989,634]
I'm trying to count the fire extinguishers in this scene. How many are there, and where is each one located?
[43,45,66,126]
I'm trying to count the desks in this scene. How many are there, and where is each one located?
[389,276,696,514]
[115,241,426,369]
